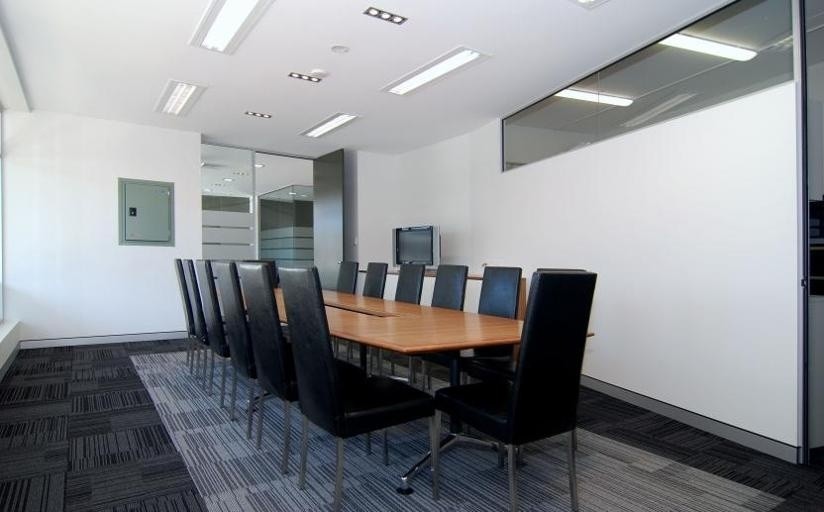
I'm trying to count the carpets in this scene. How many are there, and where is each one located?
[129,342,786,512]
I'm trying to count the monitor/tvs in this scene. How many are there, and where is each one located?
[391,225,441,270]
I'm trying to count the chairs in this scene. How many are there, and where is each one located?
[334,262,389,361]
[183,260,209,385]
[195,259,230,408]
[278,267,440,512]
[430,269,598,512]
[336,262,359,294]
[243,260,279,288]
[236,261,367,473]
[174,260,196,373]
[210,260,257,439]
[460,267,522,384]
[409,264,469,391]
[370,263,426,377]
[473,268,585,385]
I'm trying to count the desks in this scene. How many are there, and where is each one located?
[357,269,526,360]
[240,286,595,495]
